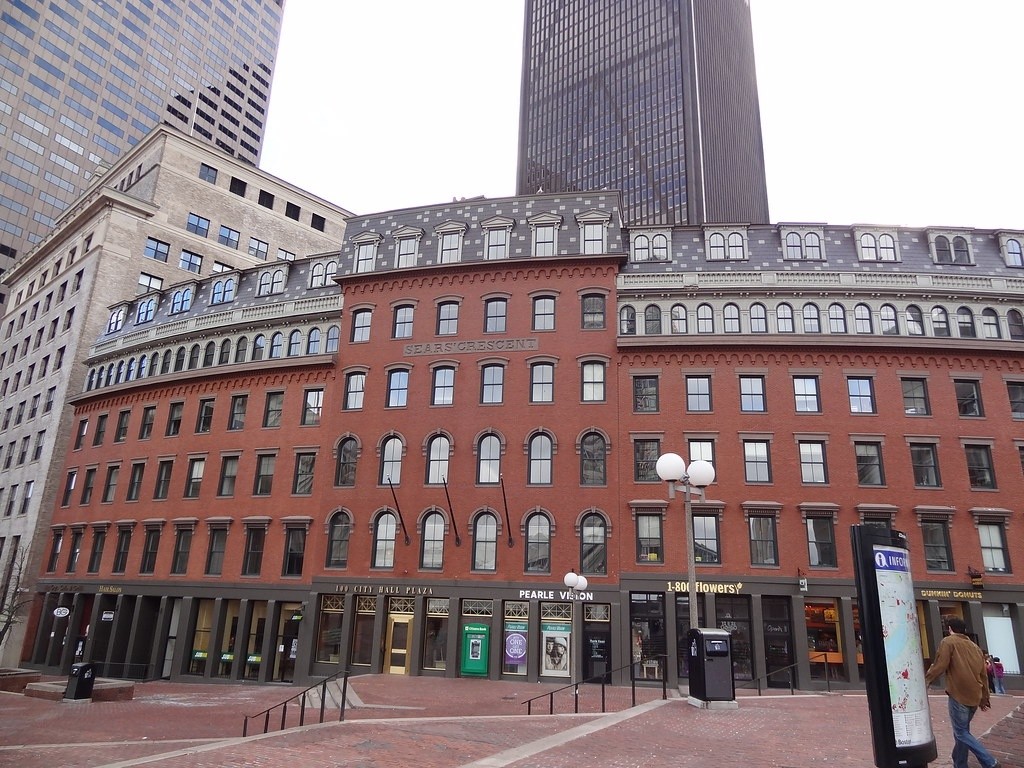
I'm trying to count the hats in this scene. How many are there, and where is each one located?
[555,638,567,648]
[546,638,554,642]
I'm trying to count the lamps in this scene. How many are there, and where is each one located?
[797,568,808,591]
[968,566,985,591]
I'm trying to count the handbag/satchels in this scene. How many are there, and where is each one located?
[986,660,994,673]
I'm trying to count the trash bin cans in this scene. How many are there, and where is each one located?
[63,662,99,700]
[685,626,737,702]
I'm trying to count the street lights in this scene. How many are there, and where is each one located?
[566,569,588,694]
[656,452,716,629]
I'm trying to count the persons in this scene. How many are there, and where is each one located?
[982,650,1005,693]
[926,618,1002,768]
[546,637,567,670]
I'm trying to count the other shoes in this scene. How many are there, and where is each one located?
[989,759,1001,768]
